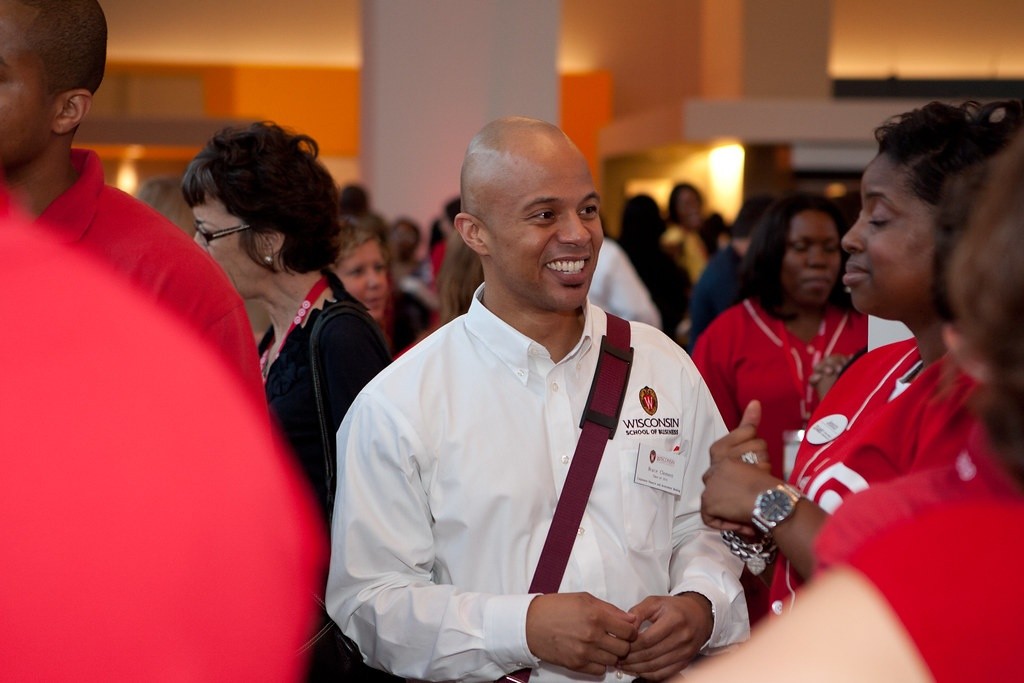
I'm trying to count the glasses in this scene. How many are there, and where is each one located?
[191,215,257,246]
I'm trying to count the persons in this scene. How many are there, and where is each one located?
[0,204,328,682]
[328,223,434,361]
[324,115,750,682]
[618,194,690,341]
[417,233,485,345]
[691,192,868,482]
[1,0,268,432]
[699,101,976,616]
[660,183,723,284]
[136,173,197,239]
[587,216,662,331]
[689,196,774,358]
[673,133,1024,683]
[339,186,462,356]
[184,120,392,682]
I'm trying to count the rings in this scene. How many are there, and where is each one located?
[741,451,758,464]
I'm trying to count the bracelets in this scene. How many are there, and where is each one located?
[721,531,778,575]
[712,606,714,616]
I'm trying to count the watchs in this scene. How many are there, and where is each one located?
[750,484,805,542]
